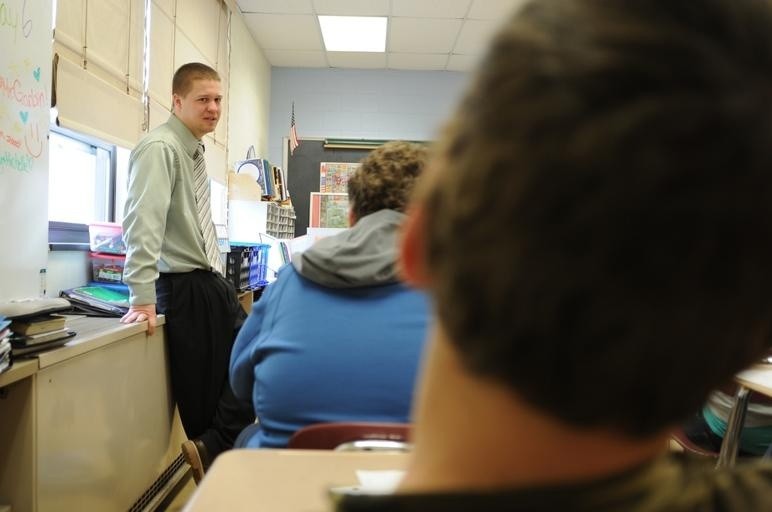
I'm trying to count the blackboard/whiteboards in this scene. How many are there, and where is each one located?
[282,138,434,238]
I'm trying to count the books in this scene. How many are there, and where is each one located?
[0,298,77,375]
[233,158,288,202]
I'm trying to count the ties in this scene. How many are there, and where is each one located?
[193,143,227,278]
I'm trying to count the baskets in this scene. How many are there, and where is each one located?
[227,242,272,291]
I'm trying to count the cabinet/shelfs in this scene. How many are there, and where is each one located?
[0,288,253,512]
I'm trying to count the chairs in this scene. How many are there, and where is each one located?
[128,442,204,512]
[290,423,412,451]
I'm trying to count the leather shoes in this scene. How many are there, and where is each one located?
[183,438,211,486]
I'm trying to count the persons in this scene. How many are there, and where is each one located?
[229,141,432,449]
[678,388,772,453]
[119,62,253,486]
[325,0,772,511]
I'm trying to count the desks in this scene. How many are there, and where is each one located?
[184,447,409,512]
[714,355,772,468]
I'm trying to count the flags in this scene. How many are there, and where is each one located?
[290,101,299,158]
